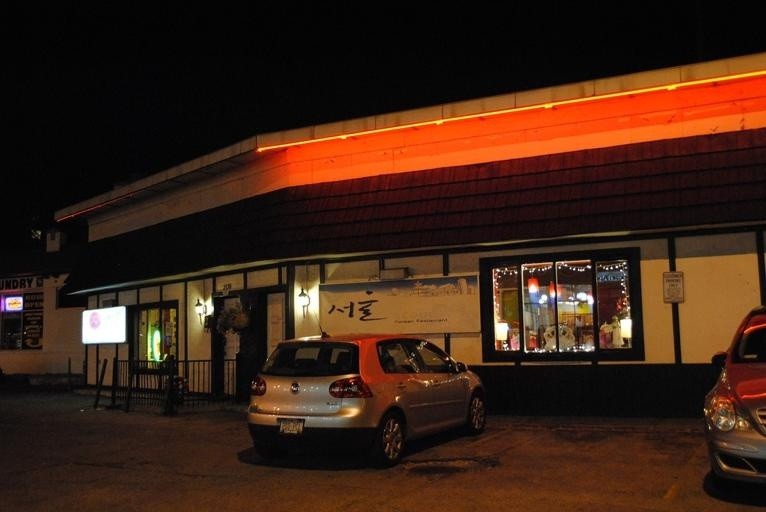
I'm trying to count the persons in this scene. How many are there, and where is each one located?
[508,314,626,351]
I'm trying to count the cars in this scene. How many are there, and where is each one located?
[246,333,487,468]
[703,305,766,484]
